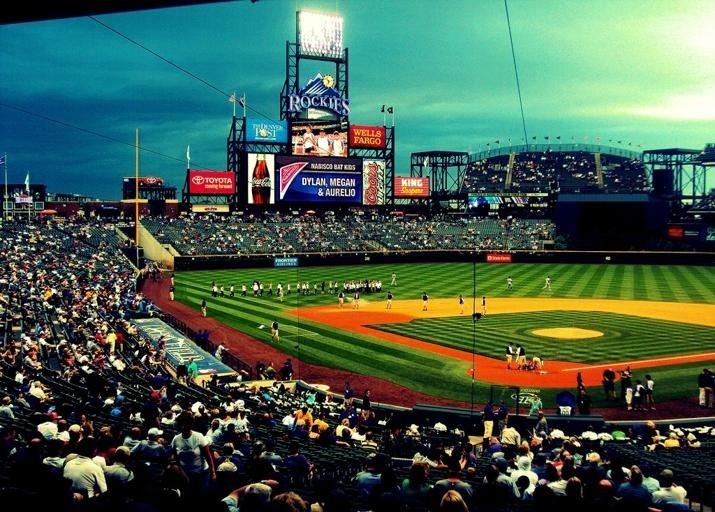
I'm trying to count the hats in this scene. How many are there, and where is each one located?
[517,456,531,471]
[68,424,84,432]
[148,427,163,435]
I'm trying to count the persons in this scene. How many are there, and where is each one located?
[303,123,315,154]
[1,209,715,511]
[330,131,345,156]
[315,128,332,156]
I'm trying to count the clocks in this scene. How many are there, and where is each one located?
[323,74,334,88]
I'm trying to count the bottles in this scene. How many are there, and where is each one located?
[252,153,271,205]
